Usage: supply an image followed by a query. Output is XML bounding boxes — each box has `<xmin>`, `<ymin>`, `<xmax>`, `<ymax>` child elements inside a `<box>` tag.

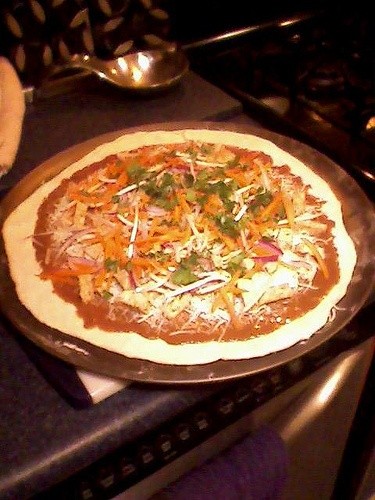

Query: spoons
<box><xmin>70</xmin><ymin>12</ymin><xmax>328</xmax><ymax>89</ymax></box>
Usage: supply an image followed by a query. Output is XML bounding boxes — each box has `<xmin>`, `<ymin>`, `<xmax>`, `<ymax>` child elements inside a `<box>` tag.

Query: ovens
<box><xmin>0</xmin><ymin>306</ymin><xmax>374</xmax><ymax>497</ymax></box>
